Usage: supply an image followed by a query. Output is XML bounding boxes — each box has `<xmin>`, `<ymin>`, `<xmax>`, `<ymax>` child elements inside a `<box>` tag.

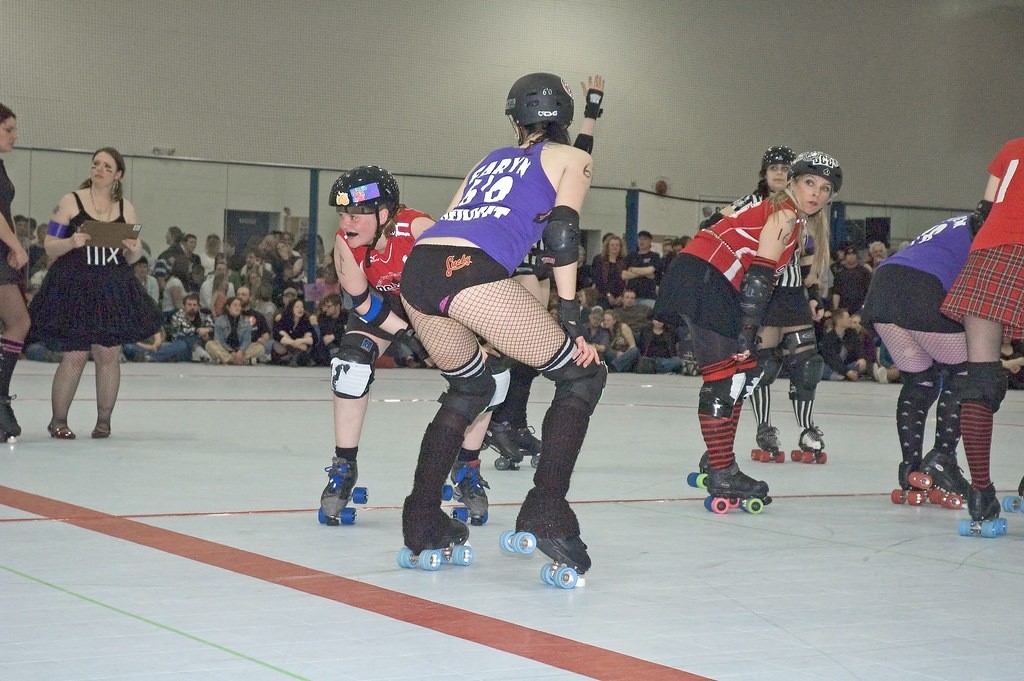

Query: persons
<box><xmin>940</xmin><ymin>138</ymin><xmax>1024</xmax><ymax>521</ymax></box>
<box><xmin>22</xmin><ymin>148</ymin><xmax>167</xmax><ymax>440</ymax></box>
<box><xmin>859</xmin><ymin>199</ymin><xmax>1024</xmax><ymax>503</ymax></box>
<box><xmin>0</xmin><ymin>103</ymin><xmax>31</xmax><ymax>443</ymax></box>
<box><xmin>650</xmin><ymin>146</ymin><xmax>842</xmax><ymax>499</ymax></box>
<box><xmin>398</xmin><ymin>73</ymin><xmax>609</xmax><ymax>575</ymax></box>
<box><xmin>13</xmin><ymin>214</ymin><xmax>1024</xmax><ymax>390</ymax></box>
<box><xmin>318</xmin><ymin>164</ymin><xmax>510</xmax><ymax>516</ymax></box>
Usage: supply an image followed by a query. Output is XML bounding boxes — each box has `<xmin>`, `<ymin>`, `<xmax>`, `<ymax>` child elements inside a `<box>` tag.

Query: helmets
<box><xmin>329</xmin><ymin>166</ymin><xmax>400</xmax><ymax>214</ymax></box>
<box><xmin>788</xmin><ymin>151</ymin><xmax>843</xmax><ymax>193</ymax></box>
<box><xmin>504</xmin><ymin>73</ymin><xmax>574</xmax><ymax>127</ymax></box>
<box><xmin>763</xmin><ymin>146</ymin><xmax>796</xmax><ymax>166</ymax></box>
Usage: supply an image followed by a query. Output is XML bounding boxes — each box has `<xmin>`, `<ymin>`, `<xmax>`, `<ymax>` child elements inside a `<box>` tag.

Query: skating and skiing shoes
<box><xmin>318</xmin><ymin>457</ymin><xmax>370</xmax><ymax>526</ymax></box>
<box><xmin>956</xmin><ymin>479</ymin><xmax>1024</xmax><ymax>537</ymax></box>
<box><xmin>498</xmin><ymin>495</ymin><xmax>593</xmax><ymax>590</ymax></box>
<box><xmin>686</xmin><ymin>451</ymin><xmax>773</xmax><ymax>514</ymax></box>
<box><xmin>750</xmin><ymin>423</ymin><xmax>785</xmax><ymax>464</ymax></box>
<box><xmin>480</xmin><ymin>419</ymin><xmax>545</xmax><ymax>471</ymax></box>
<box><xmin>442</xmin><ymin>459</ymin><xmax>491</xmax><ymax>526</ymax></box>
<box><xmin>791</xmin><ymin>427</ymin><xmax>829</xmax><ymax>465</ymax></box>
<box><xmin>398</xmin><ymin>496</ymin><xmax>476</xmax><ymax>572</ymax></box>
<box><xmin>890</xmin><ymin>449</ymin><xmax>970</xmax><ymax>511</ymax></box>
<box><xmin>0</xmin><ymin>395</ymin><xmax>22</xmax><ymax>443</ymax></box>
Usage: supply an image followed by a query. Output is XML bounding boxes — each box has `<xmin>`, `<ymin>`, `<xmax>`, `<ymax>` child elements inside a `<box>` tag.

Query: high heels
<box><xmin>47</xmin><ymin>421</ymin><xmax>76</xmax><ymax>439</ymax></box>
<box><xmin>91</xmin><ymin>420</ymin><xmax>112</xmax><ymax>438</ymax></box>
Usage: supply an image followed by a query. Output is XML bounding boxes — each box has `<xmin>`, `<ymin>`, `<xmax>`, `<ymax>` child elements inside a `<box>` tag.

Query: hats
<box><xmin>284</xmin><ymin>287</ymin><xmax>297</xmax><ymax>296</ymax></box>
<box><xmin>590</xmin><ymin>306</ymin><xmax>604</xmax><ymax>320</ymax></box>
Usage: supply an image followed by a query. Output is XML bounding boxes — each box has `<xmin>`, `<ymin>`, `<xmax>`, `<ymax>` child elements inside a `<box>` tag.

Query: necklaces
<box><xmin>90</xmin><ymin>186</ymin><xmax>113</xmax><ymax>222</ymax></box>
